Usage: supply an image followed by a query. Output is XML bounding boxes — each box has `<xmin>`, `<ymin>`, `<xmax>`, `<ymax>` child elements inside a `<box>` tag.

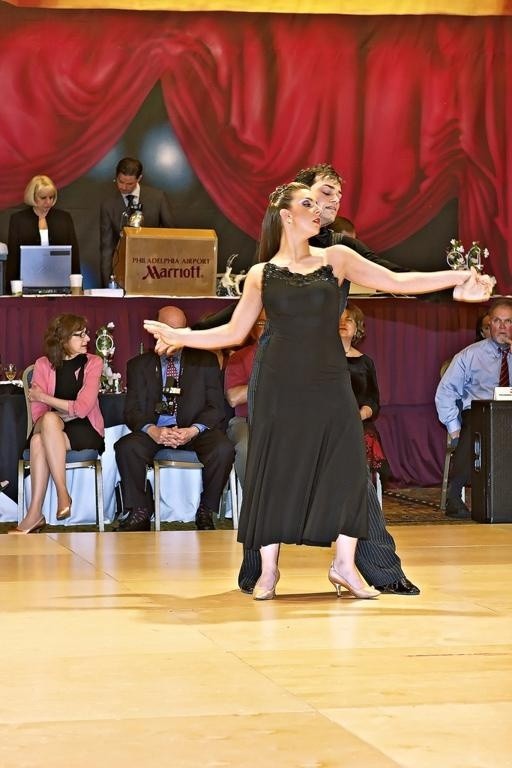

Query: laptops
<box><xmin>19</xmin><ymin>244</ymin><xmax>73</xmax><ymax>295</ymax></box>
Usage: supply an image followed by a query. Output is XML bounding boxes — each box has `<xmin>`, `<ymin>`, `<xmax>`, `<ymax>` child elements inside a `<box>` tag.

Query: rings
<box><xmin>28</xmin><ymin>394</ymin><xmax>31</xmax><ymax>399</ymax></box>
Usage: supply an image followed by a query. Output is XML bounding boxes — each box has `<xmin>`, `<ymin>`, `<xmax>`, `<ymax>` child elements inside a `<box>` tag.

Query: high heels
<box><xmin>7</xmin><ymin>514</ymin><xmax>46</xmax><ymax>535</ymax></box>
<box><xmin>56</xmin><ymin>496</ymin><xmax>72</xmax><ymax>520</ymax></box>
<box><xmin>328</xmin><ymin>560</ymin><xmax>381</xmax><ymax>599</ymax></box>
<box><xmin>252</xmin><ymin>565</ymin><xmax>280</xmax><ymax>600</ymax></box>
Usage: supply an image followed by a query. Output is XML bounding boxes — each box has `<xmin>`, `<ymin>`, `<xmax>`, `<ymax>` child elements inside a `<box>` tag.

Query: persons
<box><xmin>224</xmin><ymin>307</ymin><xmax>267</xmax><ymax>491</ymax></box>
<box><xmin>7</xmin><ymin>314</ymin><xmax>105</xmax><ymax>534</ymax></box>
<box><xmin>99</xmin><ymin>157</ymin><xmax>176</xmax><ymax>288</ymax></box>
<box><xmin>5</xmin><ymin>174</ymin><xmax>81</xmax><ymax>295</ymax></box>
<box><xmin>475</xmin><ymin>312</ymin><xmax>491</xmax><ymax>342</ymax></box>
<box><xmin>434</xmin><ymin>299</ymin><xmax>512</xmax><ymax>518</ymax></box>
<box><xmin>326</xmin><ymin>215</ymin><xmax>357</xmax><ymax>239</ymax></box>
<box><xmin>338</xmin><ymin>301</ymin><xmax>387</xmax><ymax>471</ymax></box>
<box><xmin>113</xmin><ymin>305</ymin><xmax>234</xmax><ymax>532</ymax></box>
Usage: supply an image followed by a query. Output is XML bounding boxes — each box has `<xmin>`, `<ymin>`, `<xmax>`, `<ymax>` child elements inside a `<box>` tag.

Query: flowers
<box><xmin>443</xmin><ymin>238</ymin><xmax>490</xmax><ymax>270</ymax></box>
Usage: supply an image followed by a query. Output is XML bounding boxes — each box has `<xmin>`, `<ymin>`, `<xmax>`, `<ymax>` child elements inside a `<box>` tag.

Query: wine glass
<box><xmin>3</xmin><ymin>362</ymin><xmax>18</xmax><ymax>382</ymax></box>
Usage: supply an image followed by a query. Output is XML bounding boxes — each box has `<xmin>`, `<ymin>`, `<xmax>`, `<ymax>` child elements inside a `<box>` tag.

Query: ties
<box><xmin>165</xmin><ymin>355</ymin><xmax>179</xmax><ymax>417</ymax></box>
<box><xmin>498</xmin><ymin>348</ymin><xmax>510</xmax><ymax>387</ymax></box>
<box><xmin>126</xmin><ymin>195</ymin><xmax>134</xmax><ymax>207</ymax></box>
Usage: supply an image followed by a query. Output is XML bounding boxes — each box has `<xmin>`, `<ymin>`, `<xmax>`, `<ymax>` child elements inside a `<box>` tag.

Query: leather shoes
<box><xmin>195</xmin><ymin>504</ymin><xmax>214</xmax><ymax>530</ymax></box>
<box><xmin>376</xmin><ymin>576</ymin><xmax>420</xmax><ymax>595</ymax></box>
<box><xmin>113</xmin><ymin>507</ymin><xmax>151</xmax><ymax>531</ymax></box>
<box><xmin>445</xmin><ymin>499</ymin><xmax>471</xmax><ymax>517</ymax></box>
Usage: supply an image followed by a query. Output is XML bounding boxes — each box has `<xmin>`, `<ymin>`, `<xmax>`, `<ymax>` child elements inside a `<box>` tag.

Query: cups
<box><xmin>68</xmin><ymin>273</ymin><xmax>84</xmax><ymax>296</ymax></box>
<box><xmin>10</xmin><ymin>280</ymin><xmax>23</xmax><ymax>297</ymax></box>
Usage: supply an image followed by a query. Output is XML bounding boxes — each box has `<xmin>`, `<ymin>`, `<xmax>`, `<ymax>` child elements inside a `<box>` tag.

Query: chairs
<box><xmin>440</xmin><ymin>360</ymin><xmax>459</xmax><ymax>510</ymax></box>
<box><xmin>18</xmin><ymin>365</ymin><xmax>104</xmax><ymax>531</ymax></box>
<box><xmin>144</xmin><ymin>450</ymin><xmax>239</xmax><ymax>530</ymax></box>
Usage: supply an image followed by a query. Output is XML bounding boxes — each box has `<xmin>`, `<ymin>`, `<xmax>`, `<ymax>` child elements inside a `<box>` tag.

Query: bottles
<box><xmin>108</xmin><ymin>274</ymin><xmax>118</xmax><ymax>289</ymax></box>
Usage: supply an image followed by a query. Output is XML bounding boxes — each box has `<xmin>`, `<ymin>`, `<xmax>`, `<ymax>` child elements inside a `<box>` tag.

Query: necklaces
<box><xmin>345</xmin><ymin>345</ymin><xmax>351</xmax><ymax>354</ymax></box>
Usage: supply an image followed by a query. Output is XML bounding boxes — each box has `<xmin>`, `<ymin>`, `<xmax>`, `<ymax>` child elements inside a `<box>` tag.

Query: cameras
<box><xmin>154</xmin><ymin>377</ymin><xmax>182</xmax><ymax>416</ymax></box>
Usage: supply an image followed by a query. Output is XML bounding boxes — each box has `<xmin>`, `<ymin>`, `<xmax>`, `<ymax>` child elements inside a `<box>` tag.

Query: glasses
<box><xmin>73</xmin><ymin>330</ymin><xmax>91</xmax><ymax>338</ymax></box>
